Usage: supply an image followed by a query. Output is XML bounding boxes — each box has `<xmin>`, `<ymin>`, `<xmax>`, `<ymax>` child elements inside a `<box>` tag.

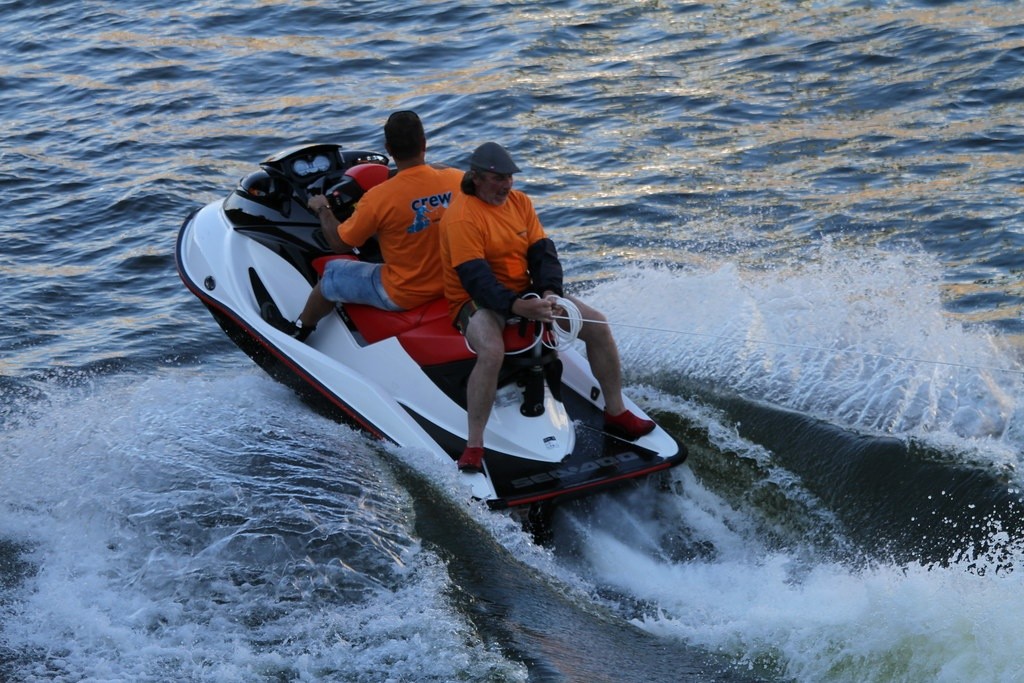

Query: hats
<box><xmin>471</xmin><ymin>142</ymin><xmax>522</xmax><ymax>174</ymax></box>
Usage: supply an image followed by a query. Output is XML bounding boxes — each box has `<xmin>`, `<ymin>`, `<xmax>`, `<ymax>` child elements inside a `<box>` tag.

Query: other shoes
<box><xmin>261</xmin><ymin>302</ymin><xmax>289</xmax><ymax>335</ymax></box>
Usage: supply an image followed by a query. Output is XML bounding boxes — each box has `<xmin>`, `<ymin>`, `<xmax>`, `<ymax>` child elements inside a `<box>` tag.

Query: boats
<box><xmin>175</xmin><ymin>142</ymin><xmax>689</xmax><ymax>511</ymax></box>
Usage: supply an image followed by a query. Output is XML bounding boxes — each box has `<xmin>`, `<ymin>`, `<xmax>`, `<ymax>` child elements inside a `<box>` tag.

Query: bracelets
<box><xmin>315</xmin><ymin>204</ymin><xmax>332</xmax><ymax>213</ymax></box>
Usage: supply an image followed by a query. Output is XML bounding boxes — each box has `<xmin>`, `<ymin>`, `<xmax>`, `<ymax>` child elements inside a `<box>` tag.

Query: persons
<box><xmin>260</xmin><ymin>111</ymin><xmax>657</xmax><ymax>472</ymax></box>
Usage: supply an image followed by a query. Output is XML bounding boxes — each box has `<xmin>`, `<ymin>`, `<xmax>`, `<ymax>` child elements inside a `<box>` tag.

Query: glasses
<box><xmin>388</xmin><ymin>110</ymin><xmax>419</xmax><ymax>121</ymax></box>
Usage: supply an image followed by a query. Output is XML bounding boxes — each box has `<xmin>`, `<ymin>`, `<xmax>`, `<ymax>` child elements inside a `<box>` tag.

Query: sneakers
<box><xmin>602</xmin><ymin>406</ymin><xmax>656</xmax><ymax>437</ymax></box>
<box><xmin>458</xmin><ymin>445</ymin><xmax>485</xmax><ymax>470</ymax></box>
